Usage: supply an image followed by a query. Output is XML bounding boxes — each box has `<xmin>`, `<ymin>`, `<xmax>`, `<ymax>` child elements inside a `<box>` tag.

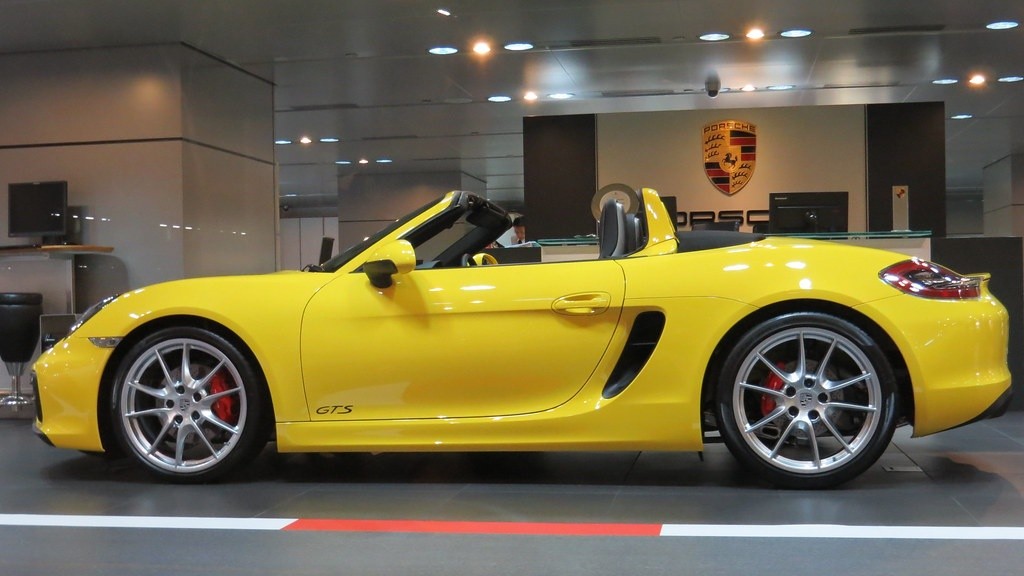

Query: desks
<box><xmin>0</xmin><ymin>245</ymin><xmax>114</xmax><ymax>314</ymax></box>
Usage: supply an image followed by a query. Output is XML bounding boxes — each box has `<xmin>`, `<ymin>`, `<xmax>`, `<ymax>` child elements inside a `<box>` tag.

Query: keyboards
<box><xmin>0</xmin><ymin>242</ymin><xmax>41</xmax><ymax>250</ymax></box>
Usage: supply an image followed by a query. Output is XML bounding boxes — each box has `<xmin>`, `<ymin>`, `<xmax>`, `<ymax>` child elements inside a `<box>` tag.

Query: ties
<box><xmin>518</xmin><ymin>240</ymin><xmax>522</xmax><ymax>244</ymax></box>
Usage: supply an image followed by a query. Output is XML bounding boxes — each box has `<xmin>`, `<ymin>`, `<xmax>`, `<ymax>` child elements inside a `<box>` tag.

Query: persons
<box><xmin>510</xmin><ymin>216</ymin><xmax>526</xmax><ymax>245</ymax></box>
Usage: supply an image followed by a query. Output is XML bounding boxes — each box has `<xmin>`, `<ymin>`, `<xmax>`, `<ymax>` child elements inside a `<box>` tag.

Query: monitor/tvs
<box><xmin>768</xmin><ymin>191</ymin><xmax>849</xmax><ymax>235</ymax></box>
<box><xmin>7</xmin><ymin>180</ymin><xmax>68</xmax><ymax>245</ymax></box>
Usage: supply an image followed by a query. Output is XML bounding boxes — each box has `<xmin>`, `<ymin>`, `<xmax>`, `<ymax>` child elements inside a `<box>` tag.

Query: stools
<box><xmin>0</xmin><ymin>292</ymin><xmax>44</xmax><ymax>412</ymax></box>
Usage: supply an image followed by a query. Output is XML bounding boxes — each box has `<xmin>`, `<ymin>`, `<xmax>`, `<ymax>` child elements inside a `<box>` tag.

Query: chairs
<box><xmin>625</xmin><ymin>213</ymin><xmax>644</xmax><ymax>254</ymax></box>
<box><xmin>597</xmin><ymin>198</ymin><xmax>629</xmax><ymax>258</ymax></box>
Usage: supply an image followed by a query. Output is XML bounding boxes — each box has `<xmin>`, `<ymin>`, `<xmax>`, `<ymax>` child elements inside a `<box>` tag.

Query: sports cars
<box><xmin>25</xmin><ymin>186</ymin><xmax>1014</xmax><ymax>492</ymax></box>
